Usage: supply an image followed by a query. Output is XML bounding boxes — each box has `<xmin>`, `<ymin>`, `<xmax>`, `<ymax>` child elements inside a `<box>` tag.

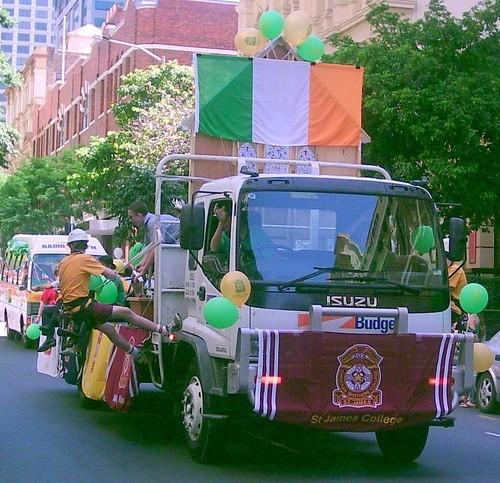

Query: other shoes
<box><xmin>57</xmin><ymin>328</ymin><xmax>89</xmax><ymax>359</ymax></box>
<box><xmin>37</xmin><ymin>338</ymin><xmax>55</xmax><ymax>352</ymax></box>
<box><xmin>39</xmin><ymin>326</ymin><xmax>54</xmax><ymax>338</ymax></box>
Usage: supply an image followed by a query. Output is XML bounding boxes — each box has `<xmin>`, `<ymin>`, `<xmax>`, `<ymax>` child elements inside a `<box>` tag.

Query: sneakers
<box><xmin>166</xmin><ymin>312</ymin><xmax>182</xmax><ymax>337</ymax></box>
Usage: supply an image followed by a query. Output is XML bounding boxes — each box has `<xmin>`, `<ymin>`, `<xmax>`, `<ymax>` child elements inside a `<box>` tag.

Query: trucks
<box><xmin>52</xmin><ymin>153</ymin><xmax>477</xmax><ymax>466</ymax></box>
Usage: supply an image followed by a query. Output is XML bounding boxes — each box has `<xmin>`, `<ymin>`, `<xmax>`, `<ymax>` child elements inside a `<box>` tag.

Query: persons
<box><xmin>448</xmin><ymin>264</ymin><xmax>468</xmax><ymax>326</ymax></box>
<box><xmin>127</xmin><ymin>201</ymin><xmax>182</xmax><ymax>282</ymax></box>
<box><xmin>22</xmin><ymin>267</ymin><xmax>47</xmax><ymax>285</ymax></box>
<box><xmin>210</xmin><ymin>209</ymin><xmax>259</xmax><ymax>267</ymax></box>
<box><xmin>35</xmin><ymin>228</ymin><xmax>183</xmax><ymax>363</ymax></box>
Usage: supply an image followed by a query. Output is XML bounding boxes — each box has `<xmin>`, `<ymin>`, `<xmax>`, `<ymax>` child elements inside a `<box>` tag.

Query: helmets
<box><xmin>67</xmin><ymin>229</ymin><xmax>89</xmax><ymax>244</ymax></box>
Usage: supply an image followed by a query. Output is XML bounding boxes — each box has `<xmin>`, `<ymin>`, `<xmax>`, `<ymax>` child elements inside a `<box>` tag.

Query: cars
<box><xmin>476</xmin><ymin>329</ymin><xmax>500</xmax><ymax>413</ymax></box>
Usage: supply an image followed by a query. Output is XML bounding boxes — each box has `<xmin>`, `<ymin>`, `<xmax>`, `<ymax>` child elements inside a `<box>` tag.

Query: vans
<box><xmin>1</xmin><ymin>234</ymin><xmax>111</xmax><ymax>348</ymax></box>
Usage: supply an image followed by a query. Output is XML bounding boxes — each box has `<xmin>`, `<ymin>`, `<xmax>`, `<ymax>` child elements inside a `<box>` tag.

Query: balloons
<box><xmin>283</xmin><ymin>10</ymin><xmax>312</xmax><ymax>46</ymax></box>
<box><xmin>259</xmin><ymin>10</ymin><xmax>284</xmax><ymax>40</ymax></box>
<box><xmin>297</xmin><ymin>36</ymin><xmax>324</xmax><ymax>61</ymax></box>
<box><xmin>114</xmin><ymin>248</ymin><xmax>123</xmax><ymax>259</ymax></box>
<box><xmin>26</xmin><ymin>324</ymin><xmax>41</xmax><ymax>340</ymax></box>
<box><xmin>459</xmin><ymin>283</ymin><xmax>488</xmax><ymax>314</ymax></box>
<box><xmin>234</xmin><ymin>28</ymin><xmax>267</xmax><ymax>56</ymax></box>
<box><xmin>220</xmin><ymin>270</ymin><xmax>251</xmax><ymax>309</ymax></box>
<box><xmin>129</xmin><ymin>243</ymin><xmax>146</xmax><ymax>265</ymax></box>
<box><xmin>409</xmin><ymin>226</ymin><xmax>435</xmax><ymax>255</ymax></box>
<box><xmin>203</xmin><ymin>298</ymin><xmax>239</xmax><ymax>329</ymax></box>
<box><xmin>96</xmin><ymin>280</ymin><xmax>118</xmax><ymax>304</ymax></box>
<box><xmin>88</xmin><ymin>275</ymin><xmax>103</xmax><ymax>295</ymax></box>
<box><xmin>474</xmin><ymin>342</ymin><xmax>495</xmax><ymax>372</ymax></box>
<box><xmin>7</xmin><ymin>239</ymin><xmax>30</xmax><ymax>256</ymax></box>
